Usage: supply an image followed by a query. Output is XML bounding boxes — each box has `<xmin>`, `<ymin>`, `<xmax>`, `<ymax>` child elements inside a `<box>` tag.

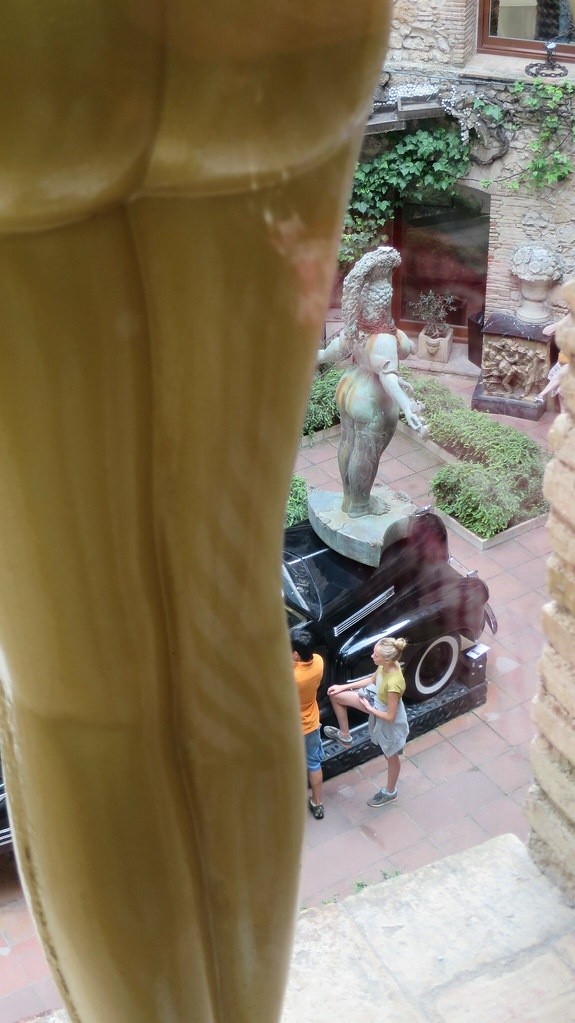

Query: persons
<box><xmin>481</xmin><ymin>339</ymin><xmax>547</xmax><ymax>398</ymax></box>
<box><xmin>318</xmin><ymin>246</ymin><xmax>423</xmax><ymax>518</ymax></box>
<box><xmin>288</xmin><ymin>628</ymin><xmax>324</xmax><ymax>819</ymax></box>
<box><xmin>324</xmin><ymin>637</ymin><xmax>409</xmax><ymax>808</ymax></box>
<box><xmin>533</xmin><ymin>361</ymin><xmax>568</xmax><ymax>414</ymax></box>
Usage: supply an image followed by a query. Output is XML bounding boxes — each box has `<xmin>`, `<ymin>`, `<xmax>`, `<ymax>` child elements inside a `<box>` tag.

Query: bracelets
<box><xmin>347</xmin><ymin>684</ymin><xmax>354</xmax><ymax>692</ymax></box>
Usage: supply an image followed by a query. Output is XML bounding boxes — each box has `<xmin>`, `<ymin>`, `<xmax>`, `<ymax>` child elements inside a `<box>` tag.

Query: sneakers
<box><xmin>324</xmin><ymin>726</ymin><xmax>354</xmax><ymax>750</ymax></box>
<box><xmin>307</xmin><ymin>796</ymin><xmax>324</xmax><ymax>819</ymax></box>
<box><xmin>367</xmin><ymin>786</ymin><xmax>398</xmax><ymax>808</ymax></box>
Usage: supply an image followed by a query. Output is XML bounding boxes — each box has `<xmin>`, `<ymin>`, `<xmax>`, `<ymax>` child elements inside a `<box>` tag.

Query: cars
<box><xmin>0</xmin><ymin>501</ymin><xmax>498</xmax><ymax>839</ymax></box>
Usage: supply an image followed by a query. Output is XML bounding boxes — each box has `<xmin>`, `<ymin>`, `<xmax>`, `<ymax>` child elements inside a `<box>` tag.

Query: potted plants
<box><xmin>408</xmin><ymin>288</ymin><xmax>461</xmax><ymax>364</ymax></box>
